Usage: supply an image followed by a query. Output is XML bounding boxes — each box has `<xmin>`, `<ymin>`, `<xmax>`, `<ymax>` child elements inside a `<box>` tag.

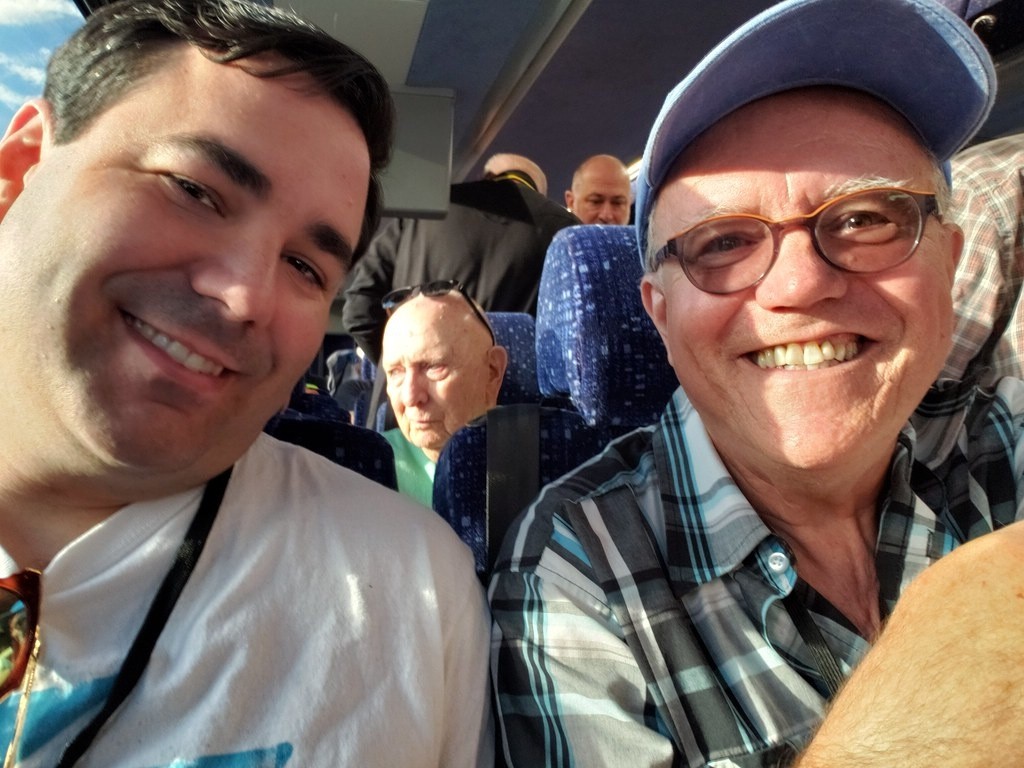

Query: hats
<box><xmin>634</xmin><ymin>0</ymin><xmax>997</xmax><ymax>270</ymax></box>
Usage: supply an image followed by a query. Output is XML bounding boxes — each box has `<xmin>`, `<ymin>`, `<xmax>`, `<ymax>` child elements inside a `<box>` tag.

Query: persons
<box><xmin>565</xmin><ymin>154</ymin><xmax>632</xmax><ymax>227</ymax></box>
<box><xmin>1</xmin><ymin>0</ymin><xmax>495</xmax><ymax>767</ymax></box>
<box><xmin>487</xmin><ymin>0</ymin><xmax>1024</xmax><ymax>767</ymax></box>
<box><xmin>343</xmin><ymin>154</ymin><xmax>583</xmax><ymax>432</ymax></box>
<box><xmin>380</xmin><ymin>291</ymin><xmax>507</xmax><ymax>507</ymax></box>
<box><xmin>304</xmin><ymin>366</ymin><xmax>374</xmax><ymax>426</ymax></box>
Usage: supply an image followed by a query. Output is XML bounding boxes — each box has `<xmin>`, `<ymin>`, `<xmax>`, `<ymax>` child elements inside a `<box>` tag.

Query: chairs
<box><xmin>269</xmin><ymin>221</ymin><xmax>676</xmax><ymax>579</ymax></box>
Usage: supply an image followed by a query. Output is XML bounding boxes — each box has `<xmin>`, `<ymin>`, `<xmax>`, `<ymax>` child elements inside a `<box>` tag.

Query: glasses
<box><xmin>0</xmin><ymin>568</ymin><xmax>42</xmax><ymax>703</ymax></box>
<box><xmin>649</xmin><ymin>186</ymin><xmax>943</xmax><ymax>296</ymax></box>
<box><xmin>382</xmin><ymin>279</ymin><xmax>495</xmax><ymax>346</ymax></box>
